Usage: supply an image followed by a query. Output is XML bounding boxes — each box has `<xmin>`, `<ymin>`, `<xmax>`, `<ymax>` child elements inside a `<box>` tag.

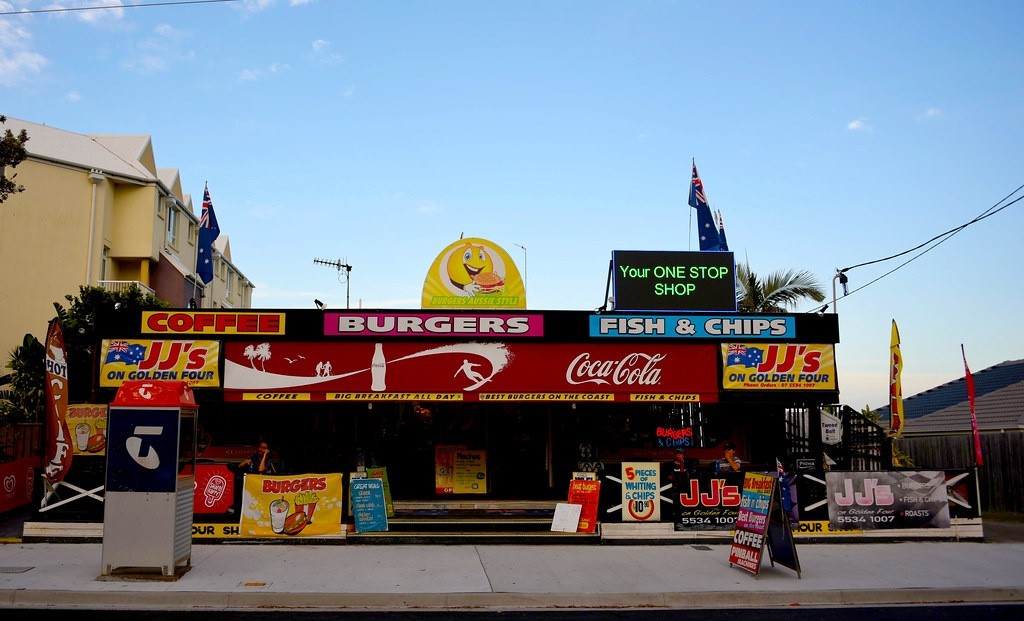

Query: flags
<box><xmin>963</xmin><ymin>349</ymin><xmax>984</xmax><ymax>464</ymax></box>
<box><xmin>686</xmin><ymin>165</ymin><xmax>729</xmax><ymax>252</ymax></box>
<box><xmin>45</xmin><ymin>321</ymin><xmax>74</xmax><ymax>481</ymax></box>
<box><xmin>889</xmin><ymin>322</ymin><xmax>902</xmax><ymax>438</ymax></box>
<box><xmin>195</xmin><ymin>188</ymin><xmax>221</xmax><ymax>285</ymax></box>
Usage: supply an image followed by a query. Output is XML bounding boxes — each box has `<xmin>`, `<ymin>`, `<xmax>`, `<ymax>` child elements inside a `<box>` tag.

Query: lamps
<box><xmin>594</xmin><ymin>305</ymin><xmax>607</xmax><ymax>315</ymax></box>
<box><xmin>816</xmin><ymin>304</ymin><xmax>829</xmax><ymax>317</ymax></box>
<box><xmin>314</xmin><ymin>299</ymin><xmax>327</xmax><ymax>310</ymax></box>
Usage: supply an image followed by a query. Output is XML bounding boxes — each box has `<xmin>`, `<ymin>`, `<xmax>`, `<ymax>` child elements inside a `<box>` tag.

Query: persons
<box><xmin>669</xmin><ymin>445</ymin><xmax>741</xmax><ymax>473</ymax></box>
<box><xmin>236</xmin><ymin>441</ymin><xmax>273</xmax><ymax>474</ymax></box>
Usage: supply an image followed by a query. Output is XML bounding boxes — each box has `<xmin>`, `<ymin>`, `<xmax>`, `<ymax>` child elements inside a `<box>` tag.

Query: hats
<box><xmin>673</xmin><ymin>445</ymin><xmax>687</xmax><ymax>455</ymax></box>
<box><xmin>722</xmin><ymin>442</ymin><xmax>737</xmax><ymax>450</ymax></box>
